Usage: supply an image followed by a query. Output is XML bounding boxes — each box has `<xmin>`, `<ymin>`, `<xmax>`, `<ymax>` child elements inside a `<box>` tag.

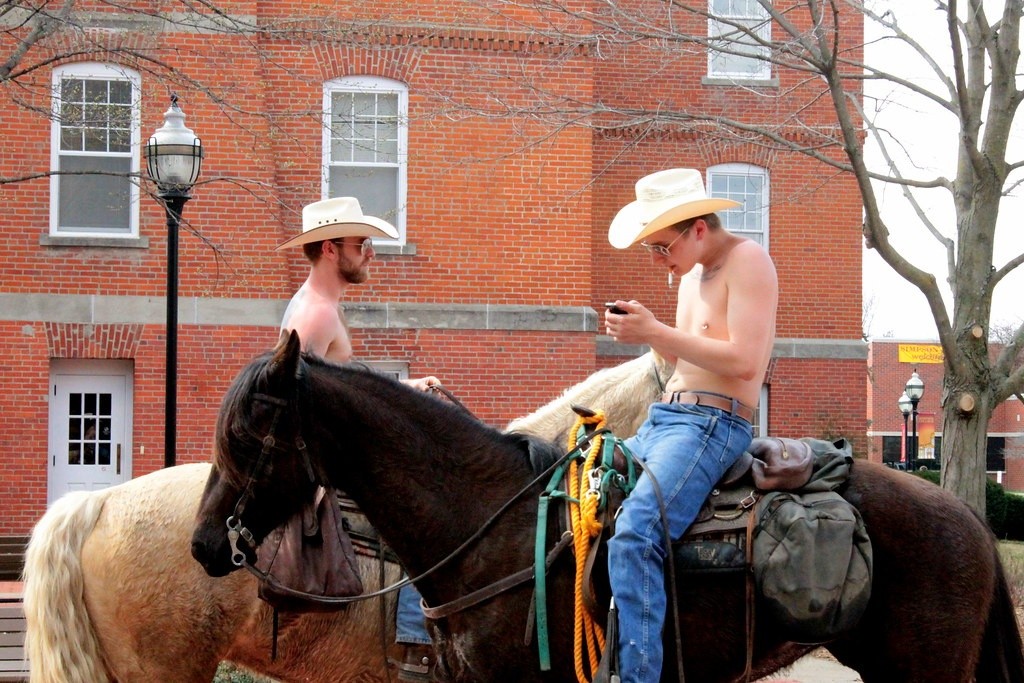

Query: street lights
<box><xmin>898</xmin><ymin>389</ymin><xmax>914</xmax><ymax>471</ymax></box>
<box><xmin>142</xmin><ymin>90</ymin><xmax>205</xmax><ymax>467</ymax></box>
<box><xmin>903</xmin><ymin>368</ymin><xmax>926</xmax><ymax>472</ymax></box>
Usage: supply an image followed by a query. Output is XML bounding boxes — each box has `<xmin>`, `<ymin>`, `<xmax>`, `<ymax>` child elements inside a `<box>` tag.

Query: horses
<box><xmin>19</xmin><ymin>342</ymin><xmax>679</xmax><ymax>682</ymax></box>
<box><xmin>190</xmin><ymin>327</ymin><xmax>1024</xmax><ymax>683</ymax></box>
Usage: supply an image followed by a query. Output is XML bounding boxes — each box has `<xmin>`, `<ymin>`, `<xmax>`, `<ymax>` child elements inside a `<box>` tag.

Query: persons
<box><xmin>275</xmin><ymin>197</ymin><xmax>445</xmax><ymax>683</ymax></box>
<box><xmin>604</xmin><ymin>168</ymin><xmax>780</xmax><ymax>683</ymax></box>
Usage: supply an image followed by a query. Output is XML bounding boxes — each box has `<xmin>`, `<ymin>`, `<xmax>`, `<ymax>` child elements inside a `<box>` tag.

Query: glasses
<box><xmin>641</xmin><ymin>227</ymin><xmax>689</xmax><ymax>256</ymax></box>
<box><xmin>335</xmin><ymin>238</ymin><xmax>372</xmax><ymax>256</ymax></box>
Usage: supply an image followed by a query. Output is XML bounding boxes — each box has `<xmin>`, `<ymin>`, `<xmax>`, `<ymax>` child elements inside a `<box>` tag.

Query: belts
<box><xmin>662</xmin><ymin>393</ymin><xmax>753</xmax><ymax>424</ymax></box>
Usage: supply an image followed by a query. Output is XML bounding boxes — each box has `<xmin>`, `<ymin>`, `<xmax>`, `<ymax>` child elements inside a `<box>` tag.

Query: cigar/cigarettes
<box><xmin>668</xmin><ymin>266</ymin><xmax>673</xmax><ymax>288</ymax></box>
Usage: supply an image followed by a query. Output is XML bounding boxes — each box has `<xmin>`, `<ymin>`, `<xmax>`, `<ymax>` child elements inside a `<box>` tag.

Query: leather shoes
<box><xmin>398</xmin><ymin>643</ymin><xmax>435</xmax><ymax>683</ymax></box>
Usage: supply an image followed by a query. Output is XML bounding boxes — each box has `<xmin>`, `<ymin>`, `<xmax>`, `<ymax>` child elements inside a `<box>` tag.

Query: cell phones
<box><xmin>605</xmin><ymin>303</ymin><xmax>628</xmax><ymax>314</ymax></box>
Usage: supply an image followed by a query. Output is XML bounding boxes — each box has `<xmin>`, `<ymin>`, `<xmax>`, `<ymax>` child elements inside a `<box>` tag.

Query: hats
<box><xmin>273</xmin><ymin>197</ymin><xmax>400</xmax><ymax>251</ymax></box>
<box><xmin>608</xmin><ymin>168</ymin><xmax>743</xmax><ymax>249</ymax></box>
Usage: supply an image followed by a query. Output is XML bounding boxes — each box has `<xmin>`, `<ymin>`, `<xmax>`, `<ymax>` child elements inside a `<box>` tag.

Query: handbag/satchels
<box><xmin>745</xmin><ymin>491</ymin><xmax>873</xmax><ymax>644</ymax></box>
<box><xmin>258</xmin><ymin>488</ymin><xmax>364</xmax><ymax>612</ymax></box>
